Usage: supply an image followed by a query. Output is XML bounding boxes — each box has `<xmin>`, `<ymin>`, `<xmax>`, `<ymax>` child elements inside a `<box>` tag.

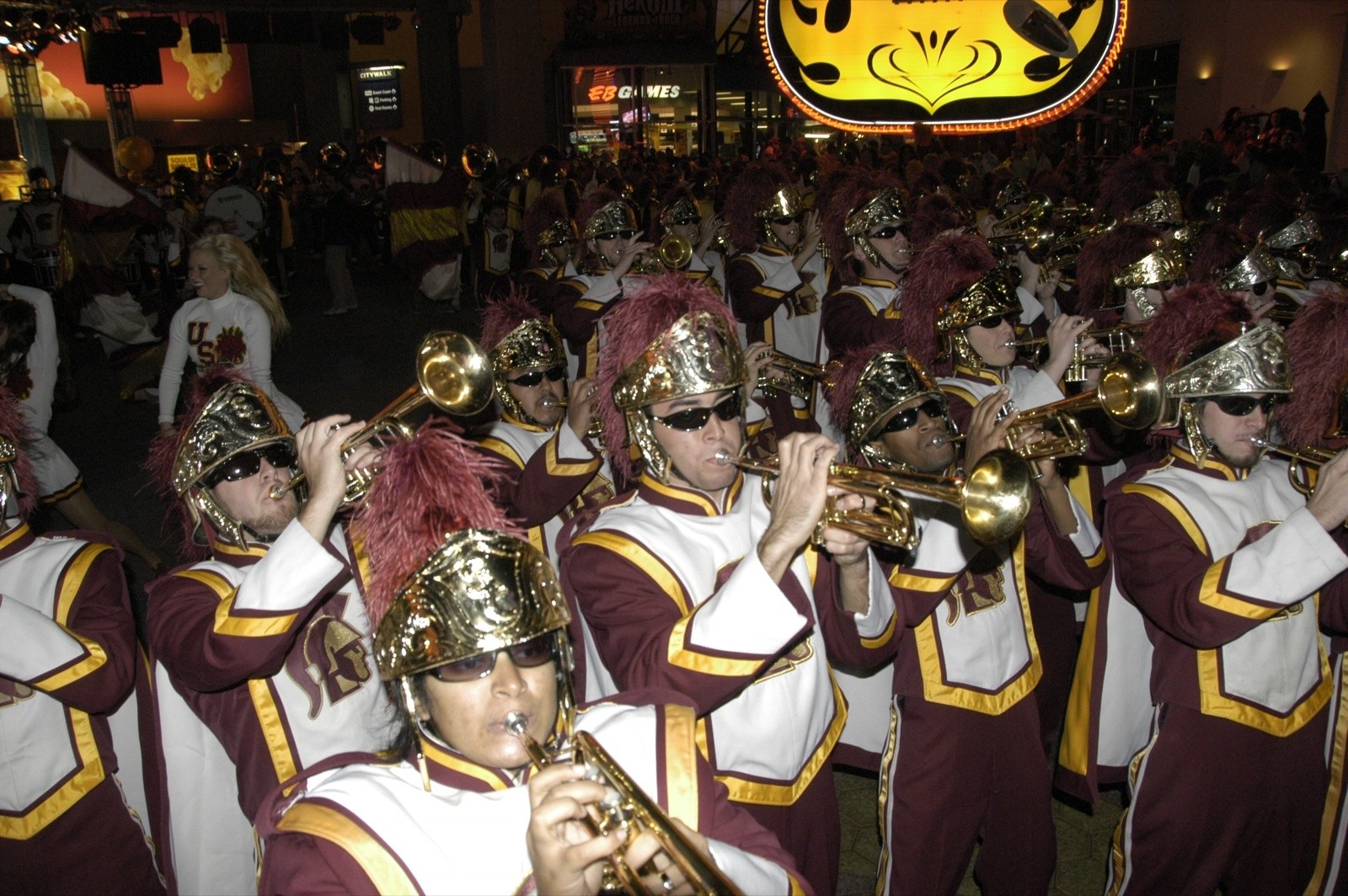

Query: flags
<box><xmin>386</xmin><ymin>144</ymin><xmax>481</xmax><ymax>304</ymax></box>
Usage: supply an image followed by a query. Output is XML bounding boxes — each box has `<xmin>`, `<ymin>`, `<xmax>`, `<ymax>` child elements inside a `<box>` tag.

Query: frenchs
<box><xmin>461</xmin><ymin>139</ymin><xmax>499</xmax><ymax>178</ymax></box>
<box><xmin>359</xmin><ymin>139</ymin><xmax>387</xmax><ymax>172</ymax></box>
<box><xmin>416</xmin><ymin>138</ymin><xmax>448</xmax><ymax>172</ymax></box>
<box><xmin>200</xmin><ymin>141</ymin><xmax>247</xmax><ymax>184</ymax></box>
<box><xmin>318</xmin><ymin>140</ymin><xmax>350</xmax><ymax>173</ymax></box>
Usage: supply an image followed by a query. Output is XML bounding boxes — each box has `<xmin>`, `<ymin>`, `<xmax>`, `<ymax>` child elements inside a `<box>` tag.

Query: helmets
<box><xmin>902</xmin><ymin>227</ymin><xmax>1025</xmax><ymax>371</ymax></box>
<box><xmin>829</xmin><ymin>342</ymin><xmax>962</xmax><ymax>473</ymax></box>
<box><xmin>350</xmin><ymin>423</ymin><xmax>570</xmax><ymax>735</ymax></box>
<box><xmin>597</xmin><ymin>269</ymin><xmax>749</xmax><ymax>484</ymax></box>
<box><xmin>1157</xmin><ymin>292</ymin><xmax>1293</xmax><ymax>469</ymax></box>
<box><xmin>528</xmin><ymin>160</ymin><xmax>806</xmax><ymax>272</ymax></box>
<box><xmin>828</xmin><ymin>169</ymin><xmax>906</xmax><ymax>269</ymax></box>
<box><xmin>484</xmin><ymin>299</ymin><xmax>569</xmax><ymax>416</ymax></box>
<box><xmin>993</xmin><ymin>157</ymin><xmax>1327</xmax><ymax>315</ymax></box>
<box><xmin>147</xmin><ymin>364</ymin><xmax>298</xmax><ymax>549</ymax></box>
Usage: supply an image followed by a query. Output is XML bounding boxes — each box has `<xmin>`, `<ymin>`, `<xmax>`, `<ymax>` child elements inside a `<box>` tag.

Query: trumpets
<box><xmin>635</xmin><ymin>232</ymin><xmax>693</xmax><ymax>272</ymax></box>
<box><xmin>1246</xmin><ymin>431</ymin><xmax>1340</xmax><ymax>498</ymax></box>
<box><xmin>503</xmin><ymin>708</ymin><xmax>745</xmax><ymax>896</ymax></box>
<box><xmin>714</xmin><ymin>351</ymin><xmax>1164</xmax><ymax>550</ymax></box>
<box><xmin>791</xmin><ymin>219</ymin><xmax>832</xmax><ymax>259</ymax></box>
<box><xmin>542</xmin><ymin>393</ymin><xmax>604</xmax><ymax>437</ymax></box>
<box><xmin>268</xmin><ymin>329</ymin><xmax>497</xmax><ymax>500</ymax></box>
<box><xmin>702</xmin><ymin>218</ymin><xmax>734</xmax><ymax>248</ymax></box>
<box><xmin>898</xmin><ymin>198</ymin><xmax>1348</xmax><ymax>383</ymax></box>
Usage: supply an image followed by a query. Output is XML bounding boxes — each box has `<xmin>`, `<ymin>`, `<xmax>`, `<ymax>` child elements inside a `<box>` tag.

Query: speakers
<box><xmin>79</xmin><ymin>32</ymin><xmax>163</xmax><ymax>85</ymax></box>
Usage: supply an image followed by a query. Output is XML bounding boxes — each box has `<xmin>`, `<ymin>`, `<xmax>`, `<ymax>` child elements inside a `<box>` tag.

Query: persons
<box><xmin>908</xmin><ymin>122</ymin><xmax>1116</xmax><ymax>762</ymax></box>
<box><xmin>0</xmin><ymin>143</ymin><xmax>385</xmax><ymax>895</ymax></box>
<box><xmin>559</xmin><ymin>275</ymin><xmax>899</xmax><ymax>896</ymax></box>
<box><xmin>407</xmin><ymin>129</ymin><xmax>911</xmax><ymax>574</ymax></box>
<box><xmin>247</xmin><ymin>415</ymin><xmax>815</xmax><ymax>895</ymax></box>
<box><xmin>833</xmin><ymin>340</ymin><xmax>1109</xmax><ymax>895</ymax></box>
<box><xmin>1055</xmin><ymin>110</ymin><xmax>1347</xmax><ymax>896</ymax></box>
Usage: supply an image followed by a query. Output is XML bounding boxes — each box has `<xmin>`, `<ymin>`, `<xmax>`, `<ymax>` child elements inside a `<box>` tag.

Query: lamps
<box><xmin>1273</xmin><ymin>67</ymin><xmax>1287</xmax><ymax>77</ymax></box>
<box><xmin>1198</xmin><ymin>76</ymin><xmax>1207</xmax><ymax>85</ymax></box>
<box><xmin>0</xmin><ymin>13</ymin><xmax>91</xmax><ymax>54</ymax></box>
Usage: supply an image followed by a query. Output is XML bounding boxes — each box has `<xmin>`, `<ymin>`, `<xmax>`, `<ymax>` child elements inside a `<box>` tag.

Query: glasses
<box><xmin>1005</xmin><ymin>196</ymin><xmax>1029</xmax><ymax>205</ymax></box>
<box><xmin>672</xmin><ymin>216</ymin><xmax>701</xmax><ymax>225</ymax></box>
<box><xmin>872</xmin><ymin>399</ymin><xmax>943</xmax><ymax>442</ymax></box>
<box><xmin>203</xmin><ymin>443</ymin><xmax>293</xmax><ymax>490</ymax></box>
<box><xmin>1158</xmin><ymin>223</ymin><xmax>1175</xmax><ymax>231</ymax></box>
<box><xmin>595</xmin><ymin>230</ymin><xmax>632</xmax><ymax>240</ymax></box>
<box><xmin>430</xmin><ymin>635</ymin><xmax>554</xmax><ymax>683</ymax></box>
<box><xmin>1202</xmin><ymin>395</ymin><xmax>1273</xmax><ymax>416</ymax></box>
<box><xmin>1252</xmin><ymin>278</ymin><xmax>1277</xmax><ymax>296</ymax></box>
<box><xmin>549</xmin><ymin>239</ymin><xmax>573</xmax><ymax>246</ymax></box>
<box><xmin>1144</xmin><ymin>278</ymin><xmax>1184</xmax><ymax>291</ymax></box>
<box><xmin>772</xmin><ymin>216</ymin><xmax>800</xmax><ymax>225</ymax></box>
<box><xmin>869</xmin><ymin>225</ymin><xmax>909</xmax><ymax>238</ymax></box>
<box><xmin>966</xmin><ymin>312</ymin><xmax>1014</xmax><ymax>330</ymax></box>
<box><xmin>647</xmin><ymin>395</ymin><xmax>737</xmax><ymax>432</ymax></box>
<box><xmin>506</xmin><ymin>368</ymin><xmax>564</xmax><ymax>386</ymax></box>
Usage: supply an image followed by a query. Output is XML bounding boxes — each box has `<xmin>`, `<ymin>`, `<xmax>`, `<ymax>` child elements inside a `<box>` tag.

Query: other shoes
<box><xmin>323</xmin><ymin>307</ymin><xmax>348</xmax><ymax>316</ymax></box>
<box><xmin>347</xmin><ymin>303</ymin><xmax>358</xmax><ymax>309</ymax></box>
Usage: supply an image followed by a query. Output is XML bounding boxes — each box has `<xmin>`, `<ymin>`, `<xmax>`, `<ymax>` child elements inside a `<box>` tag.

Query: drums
<box><xmin>202</xmin><ymin>187</ymin><xmax>268</xmax><ymax>248</ymax></box>
